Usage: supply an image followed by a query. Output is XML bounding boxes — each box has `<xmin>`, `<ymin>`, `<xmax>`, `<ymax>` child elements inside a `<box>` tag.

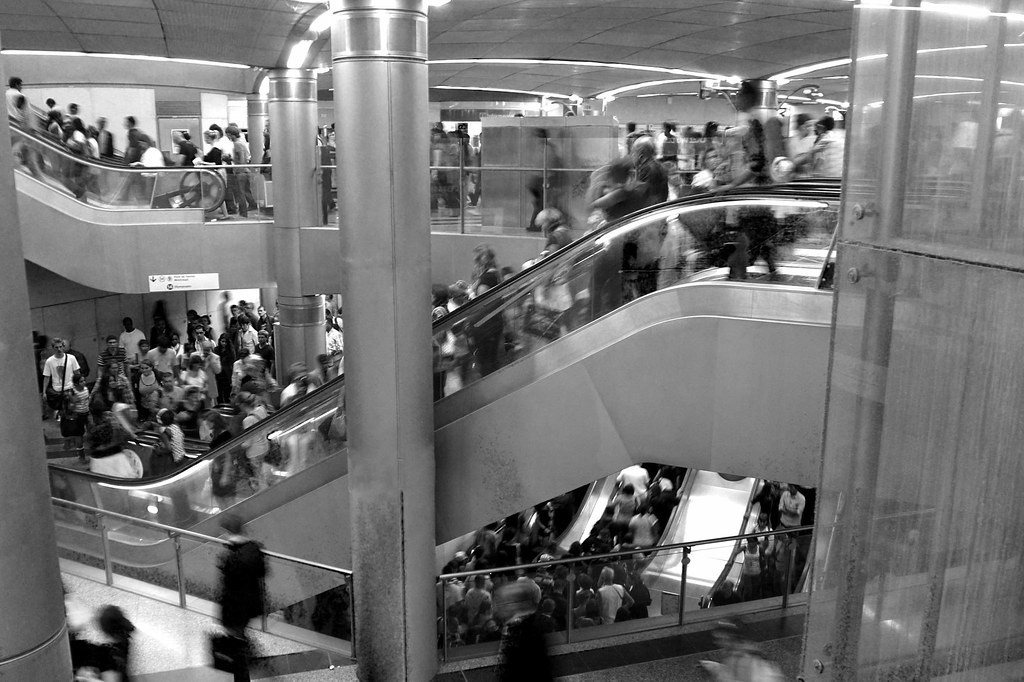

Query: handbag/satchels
<box><xmin>59</xmin><ymin>409</ymin><xmax>85</xmax><ymax>438</ymax></box>
<box><xmin>522</xmin><ymin>304</ymin><xmax>563</xmax><ymax>341</ymax></box>
<box><xmin>430</xmin><ymin>306</ymin><xmax>457</xmax><ymax>357</ymax></box>
<box><xmin>327</xmin><ymin>385</ymin><xmax>348</xmax><ymax>441</ymax></box>
<box><xmin>210</xmin><ymin>631</ymin><xmax>249</xmax><ymax>675</ymax></box>
<box><xmin>151</xmin><ymin>448</ymin><xmax>174</xmax><ymax>476</ymax></box>
<box><xmin>264</xmin><ymin>439</ymin><xmax>282</xmax><ymax>467</ymax></box>
<box><xmin>46</xmin><ymin>386</ymin><xmax>64</xmax><ymax>411</ymax></box>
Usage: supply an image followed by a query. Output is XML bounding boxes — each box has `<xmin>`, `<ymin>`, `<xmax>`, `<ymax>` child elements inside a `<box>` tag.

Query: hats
<box><xmin>535</xmin><ymin>208</ymin><xmax>564</xmax><ymax>237</ymax></box>
<box><xmin>455</xmin><ymin>551</ymin><xmax>468</xmax><ymax>561</ymax></box>
<box><xmin>771</xmin><ymin>156</ymin><xmax>798</xmax><ymax>183</ymax></box>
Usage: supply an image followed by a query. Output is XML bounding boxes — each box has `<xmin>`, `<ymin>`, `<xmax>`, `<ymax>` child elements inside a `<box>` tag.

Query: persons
<box><xmin>2</xmin><ymin>77</ymin><xmax>1024</xmax><ymax>682</ymax></box>
<box><xmin>213</xmin><ymin>514</ymin><xmax>267</xmax><ymax>682</ymax></box>
<box><xmin>490</xmin><ymin>581</ymin><xmax>553</xmax><ymax>681</ymax></box>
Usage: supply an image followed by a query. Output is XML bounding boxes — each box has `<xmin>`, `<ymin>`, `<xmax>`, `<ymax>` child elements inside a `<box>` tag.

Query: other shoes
<box><xmin>233</xmin><ymin>215</ymin><xmax>246</xmax><ymax>221</ymax></box>
<box><xmin>247</xmin><ymin>206</ymin><xmax>257</xmax><ymax>211</ymax></box>
<box><xmin>526</xmin><ymin>226</ymin><xmax>542</xmax><ymax>232</ymax></box>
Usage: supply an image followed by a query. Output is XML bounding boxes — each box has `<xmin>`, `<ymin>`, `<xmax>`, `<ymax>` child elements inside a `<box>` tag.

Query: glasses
<box><xmin>195</xmin><ymin>331</ymin><xmax>203</xmax><ymax>335</ymax></box>
